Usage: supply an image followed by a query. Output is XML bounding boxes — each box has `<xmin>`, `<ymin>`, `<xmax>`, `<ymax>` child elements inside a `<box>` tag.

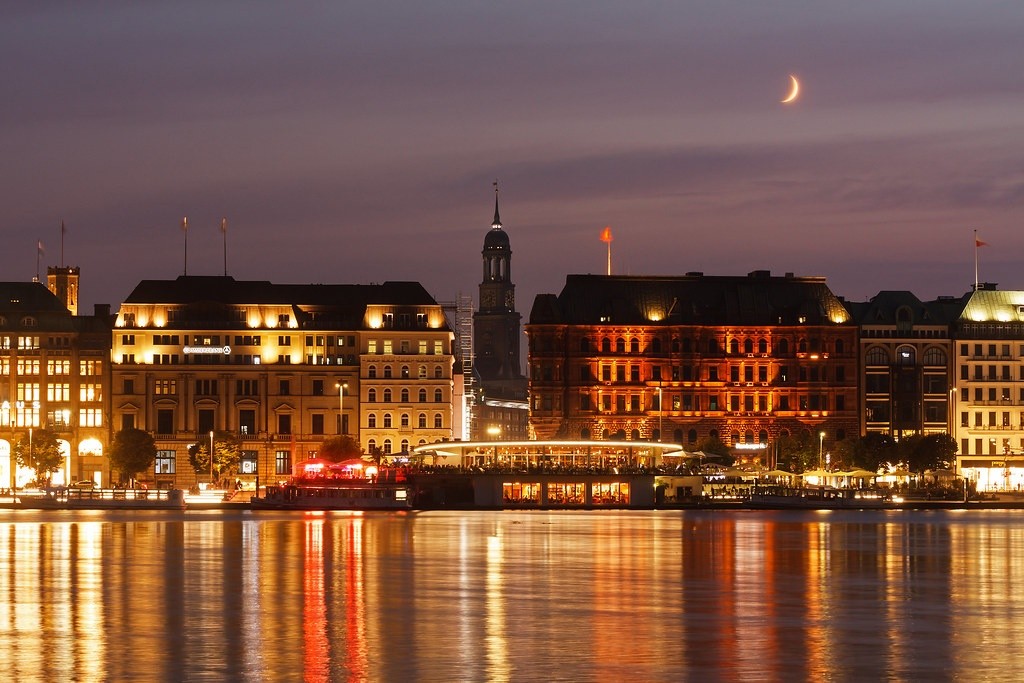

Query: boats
<box><xmin>742</xmin><ymin>485</ymin><xmax>898</xmax><ymax>509</ymax></box>
<box><xmin>249</xmin><ymin>475</ymin><xmax>417</xmax><ymax>510</ymax></box>
<box><xmin>19</xmin><ymin>488</ymin><xmax>189</xmax><ymax>510</ymax></box>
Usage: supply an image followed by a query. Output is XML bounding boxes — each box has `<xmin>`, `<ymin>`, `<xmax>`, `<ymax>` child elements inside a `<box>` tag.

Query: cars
<box><xmin>112</xmin><ymin>479</ymin><xmax>147</xmax><ymax>489</ymax></box>
<box><xmin>69</xmin><ymin>480</ymin><xmax>97</xmax><ymax>489</ymax></box>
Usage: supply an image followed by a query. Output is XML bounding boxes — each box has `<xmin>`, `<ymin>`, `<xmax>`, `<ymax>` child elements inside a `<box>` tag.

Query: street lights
<box><xmin>819</xmin><ymin>432</ymin><xmax>826</xmax><ymax>486</ymax></box>
<box><xmin>655</xmin><ymin>387</ymin><xmax>662</xmax><ymax>443</ymax></box>
<box><xmin>335</xmin><ymin>382</ymin><xmax>348</xmax><ymax>435</ymax></box>
<box><xmin>209</xmin><ymin>430</ymin><xmax>214</xmax><ymax>498</ymax></box>
<box><xmin>949</xmin><ymin>387</ymin><xmax>958</xmax><ymax>436</ymax></box>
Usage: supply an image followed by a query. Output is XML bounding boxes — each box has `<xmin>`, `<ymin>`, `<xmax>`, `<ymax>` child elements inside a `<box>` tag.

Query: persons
<box><xmin>296</xmin><ymin>470</ymin><xmax>365</xmax><ymax>479</ymax></box>
<box><xmin>590</xmin><ymin>461</ymin><xmax>658</xmax><ymax>475</ymax></box>
<box><xmin>383</xmin><ymin>458</ymin><xmax>431</xmax><ymax>475</ymax></box>
<box><xmin>503</xmin><ymin>489</ymin><xmax>540</xmax><ymax>504</ymax></box>
<box><xmin>431</xmin><ymin>464</ymin><xmax>484</xmax><ymax>474</ymax></box>
<box><xmin>592</xmin><ymin>490</ymin><xmax>628</xmax><ymax>504</ymax></box>
<box><xmin>548</xmin><ymin>490</ymin><xmax>584</xmax><ymax>505</ymax></box>
<box><xmin>529</xmin><ymin>461</ymin><xmax>587</xmax><ymax>475</ymax></box>
<box><xmin>486</xmin><ymin>463</ymin><xmax>527</xmax><ymax>474</ymax></box>
<box><xmin>659</xmin><ymin>462</ymin><xmax>720</xmax><ymax>475</ymax></box>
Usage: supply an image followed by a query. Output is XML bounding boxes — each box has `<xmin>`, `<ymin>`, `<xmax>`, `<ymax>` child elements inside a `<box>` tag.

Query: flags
<box><xmin>976</xmin><ymin>233</ymin><xmax>992</xmax><ymax>248</ymax></box>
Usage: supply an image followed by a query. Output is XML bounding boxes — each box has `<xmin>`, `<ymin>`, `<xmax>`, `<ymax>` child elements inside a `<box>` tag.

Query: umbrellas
<box><xmin>332</xmin><ymin>458</ymin><xmax>372</xmax><ymax>477</ymax></box>
<box><xmin>296</xmin><ymin>458</ymin><xmax>339</xmax><ymax>479</ymax></box>
<box><xmin>704</xmin><ymin>467</ymin><xmax>962</xmax><ymax>497</ymax></box>
<box><xmin>691</xmin><ymin>451</ymin><xmax>724</xmax><ymax>465</ymax></box>
<box><xmin>414</xmin><ymin>450</ymin><xmax>458</xmax><ymax>475</ymax></box>
<box><xmin>660</xmin><ymin>450</ymin><xmax>702</xmax><ymax>468</ymax></box>
<box><xmin>463</xmin><ymin>451</ymin><xmax>490</xmax><ymax>467</ymax></box>
